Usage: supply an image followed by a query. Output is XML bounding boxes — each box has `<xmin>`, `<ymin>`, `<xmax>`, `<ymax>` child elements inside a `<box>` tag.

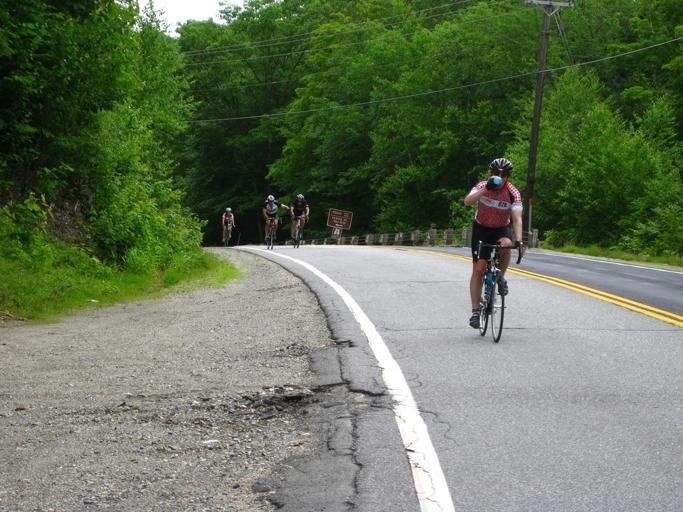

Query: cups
<box><xmin>493</xmin><ymin>177</ymin><xmax>504</xmax><ymax>187</ymax></box>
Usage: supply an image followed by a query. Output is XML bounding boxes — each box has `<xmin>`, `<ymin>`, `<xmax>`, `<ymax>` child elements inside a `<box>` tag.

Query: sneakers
<box><xmin>469</xmin><ymin>307</ymin><xmax>481</xmax><ymax>330</ymax></box>
<box><xmin>493</xmin><ymin>270</ymin><xmax>509</xmax><ymax>295</ymax></box>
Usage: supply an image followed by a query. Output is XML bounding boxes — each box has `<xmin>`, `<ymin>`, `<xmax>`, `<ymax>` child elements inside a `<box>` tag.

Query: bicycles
<box><xmin>293</xmin><ymin>214</ymin><xmax>309</xmax><ymax>248</ymax></box>
<box><xmin>266</xmin><ymin>216</ymin><xmax>283</xmax><ymax>249</ymax></box>
<box><xmin>224</xmin><ymin>221</ymin><xmax>235</xmax><ymax>247</ymax></box>
<box><xmin>474</xmin><ymin>239</ymin><xmax>522</xmax><ymax>342</ymax></box>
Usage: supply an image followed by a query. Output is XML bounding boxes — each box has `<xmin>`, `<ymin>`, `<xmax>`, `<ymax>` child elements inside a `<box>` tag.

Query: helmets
<box><xmin>226</xmin><ymin>207</ymin><xmax>232</xmax><ymax>212</ymax></box>
<box><xmin>297</xmin><ymin>194</ymin><xmax>305</xmax><ymax>201</ymax></box>
<box><xmin>268</xmin><ymin>195</ymin><xmax>275</xmax><ymax>201</ymax></box>
<box><xmin>490</xmin><ymin>159</ymin><xmax>513</xmax><ymax>175</ymax></box>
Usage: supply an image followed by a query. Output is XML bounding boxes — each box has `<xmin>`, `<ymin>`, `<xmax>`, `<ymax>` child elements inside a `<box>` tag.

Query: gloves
<box><xmin>485</xmin><ymin>177</ymin><xmax>497</xmax><ymax>191</ymax></box>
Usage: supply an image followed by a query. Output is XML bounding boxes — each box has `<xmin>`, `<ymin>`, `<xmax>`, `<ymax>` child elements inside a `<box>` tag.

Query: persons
<box><xmin>463</xmin><ymin>157</ymin><xmax>522</xmax><ymax>329</ymax></box>
<box><xmin>290</xmin><ymin>193</ymin><xmax>309</xmax><ymax>240</ymax></box>
<box><xmin>262</xmin><ymin>194</ymin><xmax>290</xmax><ymax>240</ymax></box>
<box><xmin>220</xmin><ymin>207</ymin><xmax>236</xmax><ymax>242</ymax></box>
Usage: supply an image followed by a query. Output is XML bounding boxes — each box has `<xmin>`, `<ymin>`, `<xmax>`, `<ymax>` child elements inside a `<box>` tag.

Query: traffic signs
<box><xmin>326</xmin><ymin>208</ymin><xmax>353</xmax><ymax>231</ymax></box>
